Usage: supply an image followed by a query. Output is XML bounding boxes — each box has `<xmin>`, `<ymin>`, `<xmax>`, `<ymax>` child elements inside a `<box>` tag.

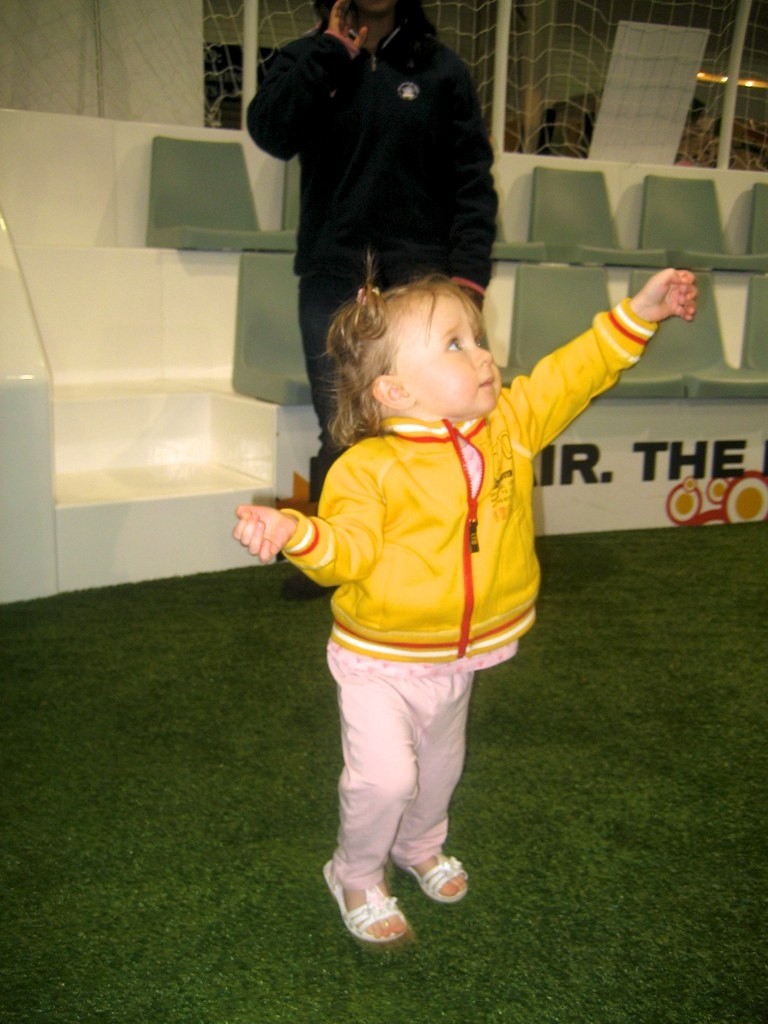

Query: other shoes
<box><xmin>282</xmin><ymin>572</ymin><xmax>336</xmax><ymax>601</ymax></box>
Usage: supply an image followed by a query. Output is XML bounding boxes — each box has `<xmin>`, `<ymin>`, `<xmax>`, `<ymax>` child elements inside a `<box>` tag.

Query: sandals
<box><xmin>323</xmin><ymin>859</ymin><xmax>407</xmax><ymax>942</ymax></box>
<box><xmin>392</xmin><ymin>852</ymin><xmax>468</xmax><ymax>903</ymax></box>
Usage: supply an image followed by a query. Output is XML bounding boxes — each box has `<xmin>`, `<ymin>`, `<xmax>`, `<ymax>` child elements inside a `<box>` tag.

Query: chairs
<box><xmin>1</xmin><ymin>108</ymin><xmax>768</xmax><ymax>603</ymax></box>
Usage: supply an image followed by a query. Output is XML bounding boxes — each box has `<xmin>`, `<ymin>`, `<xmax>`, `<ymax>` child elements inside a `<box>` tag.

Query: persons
<box><xmin>243</xmin><ymin>2</ymin><xmax>502</xmax><ymax>605</ymax></box>
<box><xmin>225</xmin><ymin>266</ymin><xmax>701</xmax><ymax>946</ymax></box>
